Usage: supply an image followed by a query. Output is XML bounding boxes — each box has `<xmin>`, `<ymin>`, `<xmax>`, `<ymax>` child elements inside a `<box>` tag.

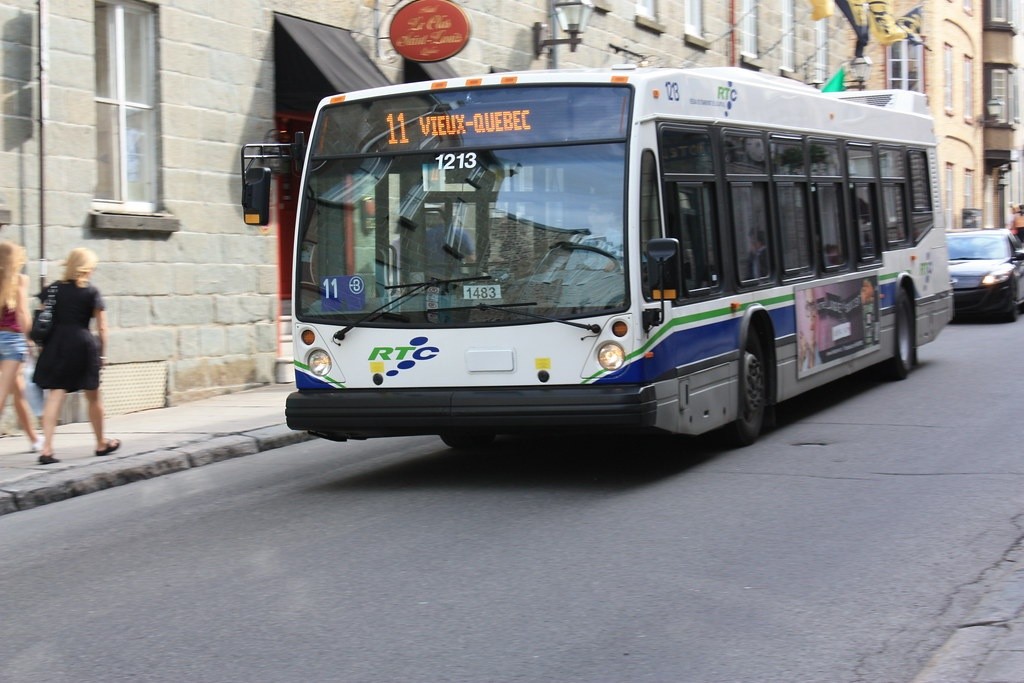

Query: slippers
<box><xmin>40</xmin><ymin>453</ymin><xmax>59</xmax><ymax>464</ymax></box>
<box><xmin>96</xmin><ymin>438</ymin><xmax>120</xmax><ymax>456</ymax></box>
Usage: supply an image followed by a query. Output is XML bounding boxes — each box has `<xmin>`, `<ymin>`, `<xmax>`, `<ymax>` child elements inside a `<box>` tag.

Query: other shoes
<box><xmin>32</xmin><ymin>436</ymin><xmax>46</xmax><ymax>452</ymax></box>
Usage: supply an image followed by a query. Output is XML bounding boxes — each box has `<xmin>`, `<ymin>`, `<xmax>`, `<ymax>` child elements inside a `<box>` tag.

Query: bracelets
<box><xmin>100</xmin><ymin>356</ymin><xmax>108</xmax><ymax>360</ymax></box>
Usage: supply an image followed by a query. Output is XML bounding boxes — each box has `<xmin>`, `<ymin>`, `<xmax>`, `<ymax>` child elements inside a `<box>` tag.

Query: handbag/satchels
<box><xmin>31</xmin><ymin>282</ymin><xmax>58</xmax><ymax>343</ymax></box>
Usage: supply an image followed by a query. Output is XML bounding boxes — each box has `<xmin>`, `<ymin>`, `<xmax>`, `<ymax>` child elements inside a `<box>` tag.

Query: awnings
<box><xmin>405</xmin><ymin>58</ymin><xmax>459</xmax><ymax>83</ymax></box>
<box><xmin>273</xmin><ymin>14</ymin><xmax>393</xmax><ymax>91</ymax></box>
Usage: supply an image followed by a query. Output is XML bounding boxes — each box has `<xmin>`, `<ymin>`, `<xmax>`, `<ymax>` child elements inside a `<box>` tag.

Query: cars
<box><xmin>945</xmin><ymin>227</ymin><xmax>1024</xmax><ymax>326</ymax></box>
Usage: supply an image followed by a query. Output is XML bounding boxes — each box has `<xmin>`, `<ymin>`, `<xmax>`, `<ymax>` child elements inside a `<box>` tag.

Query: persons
<box><xmin>390</xmin><ymin>213</ymin><xmax>476</xmax><ymax>295</ymax></box>
<box><xmin>747</xmin><ymin>228</ymin><xmax>768</xmax><ymax>279</ymax></box>
<box><xmin>799</xmin><ymin>288</ymin><xmax>822</xmax><ymax>372</ymax></box>
<box><xmin>30</xmin><ymin>246</ymin><xmax>121</xmax><ymax>463</ymax></box>
<box><xmin>565</xmin><ymin>198</ymin><xmax>647</xmax><ymax>274</ymax></box>
<box><xmin>1008</xmin><ymin>204</ymin><xmax>1024</xmax><ymax>243</ymax></box>
<box><xmin>0</xmin><ymin>241</ymin><xmax>45</xmax><ymax>453</ymax></box>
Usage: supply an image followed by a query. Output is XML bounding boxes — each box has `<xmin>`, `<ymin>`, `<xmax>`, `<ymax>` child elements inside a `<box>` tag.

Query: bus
<box><xmin>238</xmin><ymin>61</ymin><xmax>953</xmax><ymax>454</ymax></box>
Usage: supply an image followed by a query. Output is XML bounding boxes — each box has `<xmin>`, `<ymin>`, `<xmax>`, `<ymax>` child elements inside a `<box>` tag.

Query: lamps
<box><xmin>996</xmin><ymin>176</ymin><xmax>1009</xmax><ymax>192</ymax></box>
<box><xmin>532</xmin><ymin>0</ymin><xmax>593</xmax><ymax>60</ymax></box>
<box><xmin>975</xmin><ymin>95</ymin><xmax>1005</xmax><ymax>126</ymax></box>
<box><xmin>842</xmin><ymin>52</ymin><xmax>873</xmax><ymax>91</ymax></box>
